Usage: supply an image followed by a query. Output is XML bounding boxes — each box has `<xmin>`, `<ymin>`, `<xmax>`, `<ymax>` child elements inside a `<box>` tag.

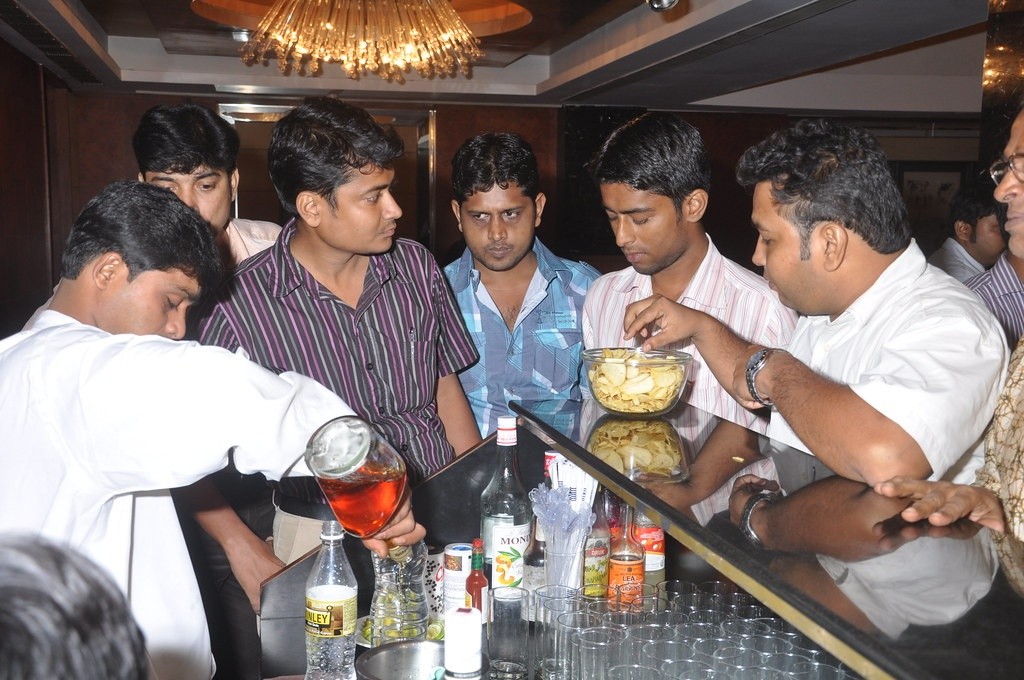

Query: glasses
<box><xmin>990</xmin><ymin>151</ymin><xmax>1024</xmax><ymax>187</ymax></box>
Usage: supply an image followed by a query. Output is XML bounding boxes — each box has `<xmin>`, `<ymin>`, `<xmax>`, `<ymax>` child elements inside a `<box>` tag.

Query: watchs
<box><xmin>738</xmin><ymin>495</ymin><xmax>770</xmax><ymax>550</ymax></box>
<box><xmin>745</xmin><ymin>347</ymin><xmax>787</xmax><ymax>407</ymax></box>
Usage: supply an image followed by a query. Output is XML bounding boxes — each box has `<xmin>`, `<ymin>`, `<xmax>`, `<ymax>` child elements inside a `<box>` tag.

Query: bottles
<box><xmin>478</xmin><ymin>415</ymin><xmax>667</xmax><ymax>633</ymax></box>
<box><xmin>466</xmin><ymin>537</ymin><xmax>489</xmax><ymax>628</ymax></box>
<box><xmin>304</xmin><ymin>416</ymin><xmax>413</xmax><ymax>564</ymax></box>
<box><xmin>303</xmin><ymin>521</ymin><xmax>358</xmax><ymax>680</ymax></box>
<box><xmin>370</xmin><ymin>540</ymin><xmax>429</xmax><ymax>650</ymax></box>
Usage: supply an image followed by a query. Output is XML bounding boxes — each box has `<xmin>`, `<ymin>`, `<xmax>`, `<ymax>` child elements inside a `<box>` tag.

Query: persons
<box><xmin>176</xmin><ymin>94</ymin><xmax>484</xmax><ymax>640</ymax></box>
<box><xmin>0</xmin><ymin>528</ymin><xmax>150</xmax><ymax>680</ymax></box>
<box><xmin>581</xmin><ymin>111</ymin><xmax>795</xmax><ymax>524</ymax></box>
<box><xmin>871</xmin><ymin>79</ymin><xmax>1024</xmax><ymax>538</ymax></box>
<box><xmin>0</xmin><ymin>180</ymin><xmax>426</xmax><ymax>680</ymax></box>
<box><xmin>621</xmin><ymin>118</ymin><xmax>1008</xmax><ymax>492</ymax></box>
<box><xmin>583</xmin><ymin>394</ymin><xmax>998</xmax><ymax>644</ymax></box>
<box><xmin>436</xmin><ymin>132</ymin><xmax>602</xmax><ymax>443</ymax></box>
<box><xmin>132</xmin><ymin>103</ymin><xmax>288</xmax><ymax>613</ymax></box>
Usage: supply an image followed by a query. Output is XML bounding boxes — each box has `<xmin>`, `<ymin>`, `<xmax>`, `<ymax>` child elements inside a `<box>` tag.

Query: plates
<box><xmin>355</xmin><ymin>612</ymin><xmax>445</xmax><ymax>649</ymax></box>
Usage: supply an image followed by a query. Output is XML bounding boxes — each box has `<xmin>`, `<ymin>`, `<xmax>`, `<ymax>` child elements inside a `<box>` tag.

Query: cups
<box><xmin>486</xmin><ymin>579</ymin><xmax>865</xmax><ymax>680</ymax></box>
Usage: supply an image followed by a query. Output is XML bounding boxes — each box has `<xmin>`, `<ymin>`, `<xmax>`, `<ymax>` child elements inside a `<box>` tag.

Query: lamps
<box><xmin>188</xmin><ymin>0</ymin><xmax>533</xmax><ymax>81</ymax></box>
<box><xmin>645</xmin><ymin>0</ymin><xmax>679</xmax><ymax>12</ymax></box>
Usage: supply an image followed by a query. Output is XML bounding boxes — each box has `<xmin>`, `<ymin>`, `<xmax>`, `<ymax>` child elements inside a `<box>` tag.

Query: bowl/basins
<box><xmin>354</xmin><ymin>640</ymin><xmax>489</xmax><ymax>680</ymax></box>
<box><xmin>583</xmin><ymin>414</ymin><xmax>689</xmax><ymax>485</ymax></box>
<box><xmin>581</xmin><ymin>347</ymin><xmax>694</xmax><ymax>420</ymax></box>
